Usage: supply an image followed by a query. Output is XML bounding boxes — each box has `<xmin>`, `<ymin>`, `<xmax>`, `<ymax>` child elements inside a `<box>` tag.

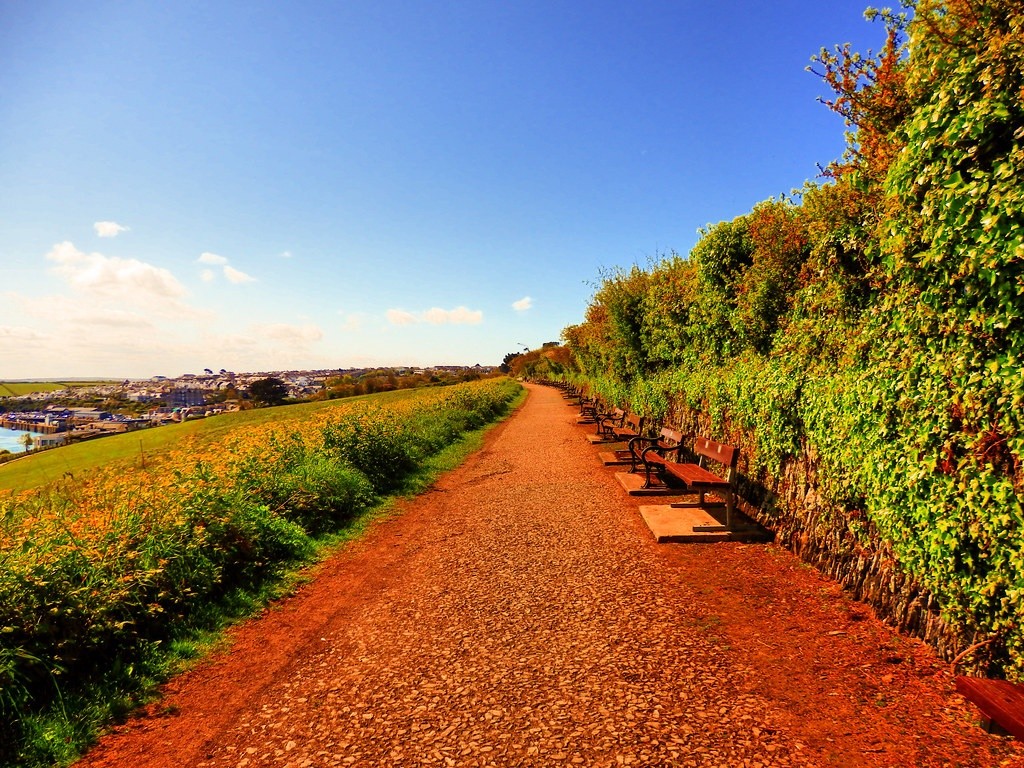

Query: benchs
<box><xmin>535</xmin><ymin>379</ymin><xmax>685</xmax><ymax>497</ymax></box>
<box><xmin>665</xmin><ymin>436</ymin><xmax>749</xmax><ymax>531</ymax></box>
